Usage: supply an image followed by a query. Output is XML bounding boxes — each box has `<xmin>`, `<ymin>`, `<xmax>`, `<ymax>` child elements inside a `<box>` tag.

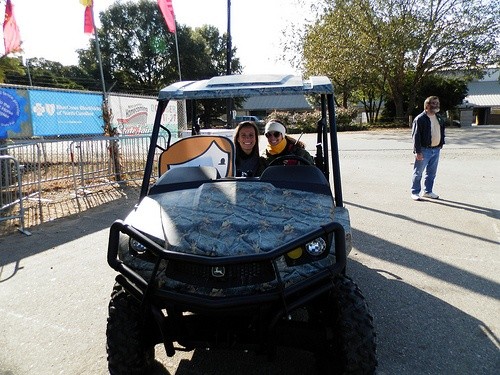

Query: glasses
<box><xmin>266</xmin><ymin>131</ymin><xmax>280</xmax><ymax>138</ymax></box>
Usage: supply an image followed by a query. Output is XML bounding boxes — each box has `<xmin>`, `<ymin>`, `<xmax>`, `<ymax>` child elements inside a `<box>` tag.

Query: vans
<box><xmin>236</xmin><ymin>115</ymin><xmax>260</xmax><ymax>126</ymax></box>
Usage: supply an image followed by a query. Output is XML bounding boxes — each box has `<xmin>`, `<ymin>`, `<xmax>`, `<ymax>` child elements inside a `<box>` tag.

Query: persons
<box><xmin>232</xmin><ymin>121</ymin><xmax>266</xmax><ymax>177</ymax></box>
<box><xmin>263</xmin><ymin>120</ymin><xmax>315</xmax><ymax>166</ymax></box>
<box><xmin>409</xmin><ymin>96</ymin><xmax>447</xmax><ymax>201</ymax></box>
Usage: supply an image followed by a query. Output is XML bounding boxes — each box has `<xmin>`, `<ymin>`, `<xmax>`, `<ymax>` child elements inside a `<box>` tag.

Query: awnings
<box><xmin>465</xmin><ymin>94</ymin><xmax>500</xmax><ymax>107</ymax></box>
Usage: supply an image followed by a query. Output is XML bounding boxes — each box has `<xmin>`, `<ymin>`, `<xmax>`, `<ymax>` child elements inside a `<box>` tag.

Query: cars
<box><xmin>210</xmin><ymin>119</ymin><xmax>228</xmax><ymax>127</ymax></box>
<box><xmin>106</xmin><ymin>74</ymin><xmax>378</xmax><ymax>375</ymax></box>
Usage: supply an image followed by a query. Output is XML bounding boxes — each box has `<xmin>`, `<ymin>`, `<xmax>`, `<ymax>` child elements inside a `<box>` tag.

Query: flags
<box><xmin>156</xmin><ymin>0</ymin><xmax>176</xmax><ymax>33</ymax></box>
<box><xmin>81</xmin><ymin>0</ymin><xmax>93</xmax><ymax>34</ymax></box>
<box><xmin>2</xmin><ymin>0</ymin><xmax>22</xmax><ymax>54</ymax></box>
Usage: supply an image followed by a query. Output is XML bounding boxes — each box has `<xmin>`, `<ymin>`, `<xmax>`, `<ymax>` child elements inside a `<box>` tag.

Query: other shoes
<box><xmin>422</xmin><ymin>192</ymin><xmax>439</xmax><ymax>199</ymax></box>
<box><xmin>410</xmin><ymin>194</ymin><xmax>422</xmax><ymax>200</ymax></box>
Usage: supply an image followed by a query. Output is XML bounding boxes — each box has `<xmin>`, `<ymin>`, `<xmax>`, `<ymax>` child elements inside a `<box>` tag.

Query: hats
<box><xmin>265</xmin><ymin>120</ymin><xmax>285</xmax><ymax>138</ymax></box>
